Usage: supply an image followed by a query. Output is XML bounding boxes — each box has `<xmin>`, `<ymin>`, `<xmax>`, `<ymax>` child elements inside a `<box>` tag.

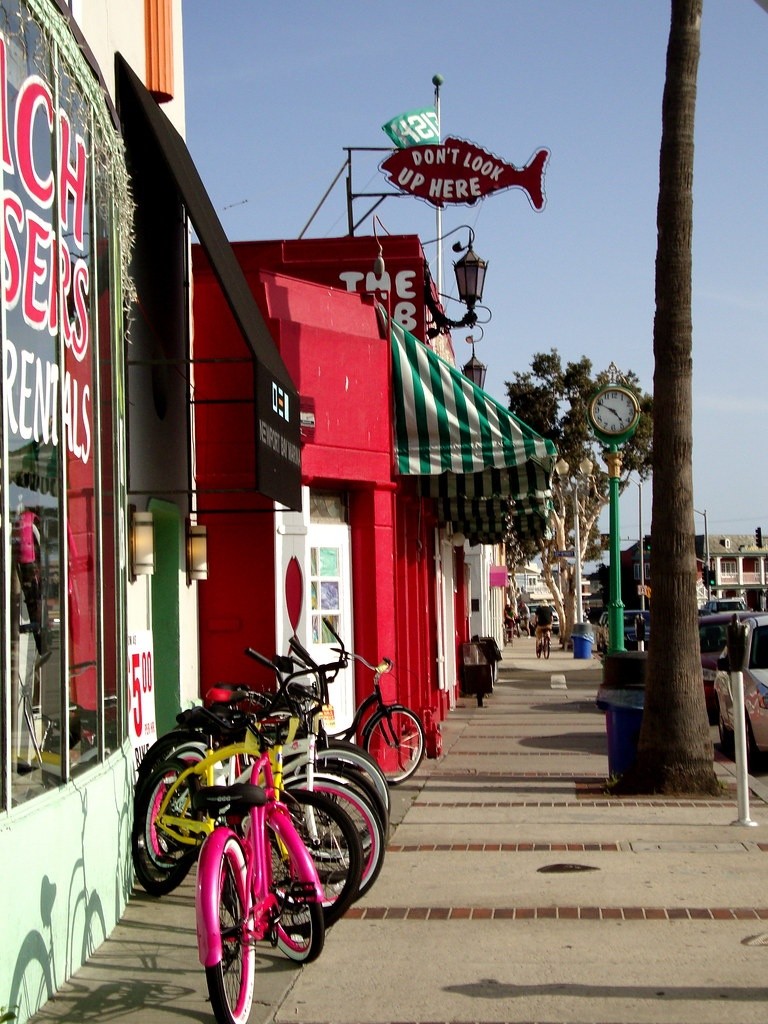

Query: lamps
<box><xmin>440</xmin><ymin>325</ymin><xmax>488</xmax><ymax>390</ymax></box>
<box><xmin>443</xmin><ymin>532</ymin><xmax>465</xmax><ymax>547</ymax></box>
<box><xmin>184</xmin><ymin>517</ymin><xmax>208</xmax><ymax>586</ymax></box>
<box><xmin>373</xmin><ymin>214</ymin><xmax>390</xmax><ymax>281</ymax></box>
<box><xmin>425</xmin><ymin>230</ymin><xmax>489</xmax><ymax>339</ymax></box>
<box><xmin>127</xmin><ymin>503</ymin><xmax>153</xmax><ymax>584</ymax></box>
<box><xmin>422</xmin><ymin>225</ymin><xmax>475</xmax><ymax>252</ymax></box>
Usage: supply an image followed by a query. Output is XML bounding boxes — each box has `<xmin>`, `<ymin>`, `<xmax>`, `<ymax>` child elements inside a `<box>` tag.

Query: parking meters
<box><xmin>716</xmin><ymin>613</ymin><xmax>758</xmax><ymax>827</ymax></box>
<box><xmin>634</xmin><ymin>613</ymin><xmax>645</xmax><ymax>652</ymax></box>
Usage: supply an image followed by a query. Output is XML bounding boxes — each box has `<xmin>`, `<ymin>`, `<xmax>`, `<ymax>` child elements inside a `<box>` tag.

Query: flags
<box><xmin>381</xmin><ymin>106</ymin><xmax>439</xmax><ymax>150</ymax></box>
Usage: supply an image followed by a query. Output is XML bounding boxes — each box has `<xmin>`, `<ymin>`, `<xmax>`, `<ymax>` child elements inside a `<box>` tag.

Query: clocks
<box><xmin>591</xmin><ymin>388</ymin><xmax>639</xmax><ymax>435</ymax></box>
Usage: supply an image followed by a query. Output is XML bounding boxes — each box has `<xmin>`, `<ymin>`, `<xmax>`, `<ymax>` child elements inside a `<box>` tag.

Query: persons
<box><xmin>503</xmin><ymin>602</ymin><xmax>532</xmax><ymax>639</ymax></box>
<box><xmin>534</xmin><ymin>600</ymin><xmax>553</xmax><ymax>647</ymax></box>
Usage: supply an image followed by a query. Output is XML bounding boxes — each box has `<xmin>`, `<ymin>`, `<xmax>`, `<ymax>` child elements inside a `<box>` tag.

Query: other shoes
<box><xmin>537</xmin><ymin>643</ymin><xmax>541</xmax><ymax>652</ymax></box>
<box><xmin>548</xmin><ymin>637</ymin><xmax>551</xmax><ymax>644</ymax></box>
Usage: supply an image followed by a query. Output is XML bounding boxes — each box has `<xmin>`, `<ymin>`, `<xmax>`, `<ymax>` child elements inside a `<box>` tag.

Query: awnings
<box><xmin>379</xmin><ymin>303</ymin><xmax>558</xmax><ymax>546</ymax></box>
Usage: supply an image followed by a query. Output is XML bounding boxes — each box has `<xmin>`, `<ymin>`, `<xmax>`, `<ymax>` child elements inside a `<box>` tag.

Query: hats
<box><xmin>539</xmin><ymin>599</ymin><xmax>549</xmax><ymax>608</ymax></box>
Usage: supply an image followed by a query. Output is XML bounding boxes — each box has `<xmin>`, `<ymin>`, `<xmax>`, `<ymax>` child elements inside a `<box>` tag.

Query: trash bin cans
<box><xmin>573</xmin><ymin>623</ymin><xmax>592</xmax><ymax>659</ymax></box>
<box><xmin>597</xmin><ymin>652</ymin><xmax>649</xmax><ymax>780</ymax></box>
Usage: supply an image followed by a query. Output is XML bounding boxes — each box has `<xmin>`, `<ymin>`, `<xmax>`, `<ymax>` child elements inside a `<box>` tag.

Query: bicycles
<box><xmin>132</xmin><ymin>618</ymin><xmax>426</xmax><ymax>1024</ymax></box>
<box><xmin>532</xmin><ymin>622</ymin><xmax>551</xmax><ymax>659</ymax></box>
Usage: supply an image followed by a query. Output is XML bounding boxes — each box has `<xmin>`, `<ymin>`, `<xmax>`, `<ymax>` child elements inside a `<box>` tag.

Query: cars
<box><xmin>526</xmin><ymin>603</ymin><xmax>559</xmax><ymax>636</ymax></box>
<box><xmin>712</xmin><ymin>616</ymin><xmax>768</xmax><ymax>771</ymax></box>
<box><xmin>596</xmin><ymin>609</ymin><xmax>650</xmax><ymax>654</ymax></box>
<box><xmin>699</xmin><ymin>611</ymin><xmax>768</xmax><ymax>712</ymax></box>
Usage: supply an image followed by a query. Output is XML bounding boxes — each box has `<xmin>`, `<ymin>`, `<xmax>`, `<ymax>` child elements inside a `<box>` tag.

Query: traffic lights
<box><xmin>701</xmin><ymin>565</ymin><xmax>709</xmax><ymax>589</ymax></box>
<box><xmin>755</xmin><ymin>527</ymin><xmax>762</xmax><ymax>548</ymax></box>
<box><xmin>709</xmin><ymin>570</ymin><xmax>718</xmax><ymax>586</ymax></box>
